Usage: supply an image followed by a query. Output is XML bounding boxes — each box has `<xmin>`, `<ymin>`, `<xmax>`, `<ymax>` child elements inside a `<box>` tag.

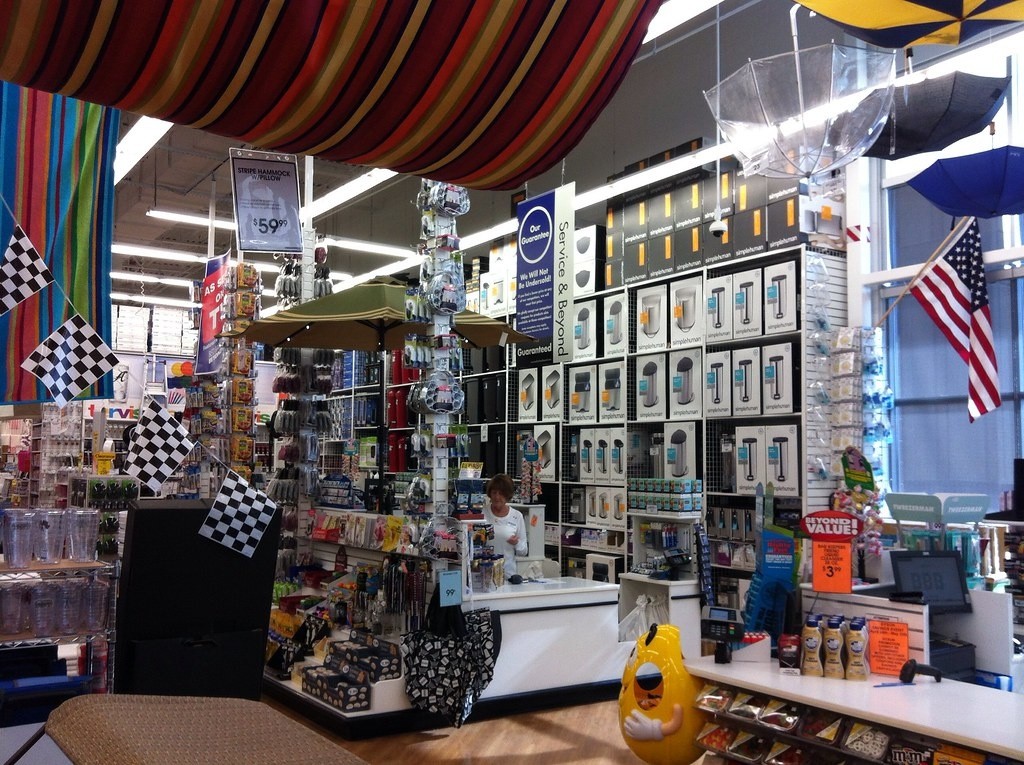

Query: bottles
<box><xmin>800</xmin><ymin>614</ymin><xmax>871</xmax><ymax>681</ymax></box>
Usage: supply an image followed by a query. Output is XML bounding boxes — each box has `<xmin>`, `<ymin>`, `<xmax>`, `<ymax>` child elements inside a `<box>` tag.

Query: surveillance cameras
<box><xmin>709</xmin><ymin>221</ymin><xmax>727</xmax><ymax>237</ymax></box>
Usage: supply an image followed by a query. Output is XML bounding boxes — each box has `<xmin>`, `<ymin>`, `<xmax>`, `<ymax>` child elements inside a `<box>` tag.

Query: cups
<box><xmin>0</xmin><ymin>508</ymin><xmax>110</xmax><ymax>636</ymax></box>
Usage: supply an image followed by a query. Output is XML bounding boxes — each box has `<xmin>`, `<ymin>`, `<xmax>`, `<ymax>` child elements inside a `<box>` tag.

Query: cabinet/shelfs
<box><xmin>26</xmin><ymin>401</ymin><xmax>85</xmax><ymax>511</ymax></box>
<box><xmin>315</xmin><ymin>146</ymin><xmax>846</xmax><ymax>585</ymax></box>
<box><xmin>85</xmin><ymin>417</ymin><xmax>139</xmax><ymax>479</ymax></box>
<box><xmin>0</xmin><ymin>556</ymin><xmax>116</xmax><ymax>727</ymax></box>
<box><xmin>253</xmin><ymin>422</ymin><xmax>274</xmax><ymax>461</ymax></box>
<box><xmin>688</xmin><ymin>651</ymin><xmax>1024</xmax><ymax>765</ymax></box>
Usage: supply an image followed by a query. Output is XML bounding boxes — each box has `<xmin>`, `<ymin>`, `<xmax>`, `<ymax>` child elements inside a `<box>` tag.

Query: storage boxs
<box><xmin>320</xmin><ymin>146</ymin><xmax>800</xmax><ymax>586</ymax></box>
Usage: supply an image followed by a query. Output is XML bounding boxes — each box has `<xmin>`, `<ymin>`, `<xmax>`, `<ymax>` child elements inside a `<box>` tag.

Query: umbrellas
<box><xmin>700</xmin><ymin>4</ymin><xmax>898</xmax><ymax>179</ymax></box>
<box><xmin>906</xmin><ymin>122</ymin><xmax>1024</xmax><ymax>217</ymax></box>
<box><xmin>792</xmin><ymin>0</ymin><xmax>1024</xmax><ymax>49</ymax></box>
<box><xmin>827</xmin><ymin>51</ymin><xmax>1012</xmax><ymax>161</ymax></box>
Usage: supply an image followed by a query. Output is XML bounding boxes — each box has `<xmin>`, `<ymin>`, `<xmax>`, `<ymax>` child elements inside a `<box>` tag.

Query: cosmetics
<box><xmin>802</xmin><ymin>612</ymin><xmax>871</xmax><ymax>680</ymax></box>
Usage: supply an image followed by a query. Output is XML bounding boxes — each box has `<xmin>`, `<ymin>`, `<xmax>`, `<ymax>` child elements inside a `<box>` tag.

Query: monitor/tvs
<box><xmin>890</xmin><ymin>550</ymin><xmax>973</xmax><ymax>614</ymax></box>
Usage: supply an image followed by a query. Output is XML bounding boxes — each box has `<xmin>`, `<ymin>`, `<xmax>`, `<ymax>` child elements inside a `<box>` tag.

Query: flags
<box><xmin>199</xmin><ymin>468</ymin><xmax>277</xmax><ymax>558</ymax></box>
<box><xmin>0</xmin><ymin>224</ymin><xmax>56</xmax><ymax>315</ymax></box>
<box><xmin>910</xmin><ymin>216</ymin><xmax>1006</xmax><ymax>424</ymax></box>
<box><xmin>20</xmin><ymin>313</ymin><xmax>122</xmax><ymax>408</ymax></box>
<box><xmin>122</xmin><ymin>398</ymin><xmax>198</xmax><ymax>493</ymax></box>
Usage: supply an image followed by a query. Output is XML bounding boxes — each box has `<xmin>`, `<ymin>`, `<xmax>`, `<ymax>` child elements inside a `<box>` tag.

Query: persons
<box><xmin>477</xmin><ymin>472</ymin><xmax>529</xmax><ymax>579</ymax></box>
